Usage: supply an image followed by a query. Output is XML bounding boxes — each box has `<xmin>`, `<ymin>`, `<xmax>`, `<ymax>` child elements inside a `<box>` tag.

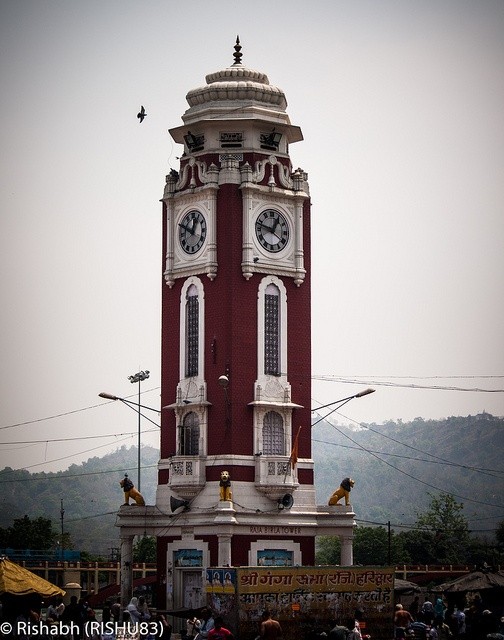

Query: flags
<box><xmin>290</xmin><ymin>425</ymin><xmax>301</xmax><ymax>470</ymax></box>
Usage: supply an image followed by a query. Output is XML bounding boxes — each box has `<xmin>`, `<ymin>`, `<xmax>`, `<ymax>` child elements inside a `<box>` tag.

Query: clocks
<box><xmin>176</xmin><ymin>209</ymin><xmax>206</xmax><ymax>255</ymax></box>
<box><xmin>254</xmin><ymin>208</ymin><xmax>289</xmax><ymax>253</ymax></box>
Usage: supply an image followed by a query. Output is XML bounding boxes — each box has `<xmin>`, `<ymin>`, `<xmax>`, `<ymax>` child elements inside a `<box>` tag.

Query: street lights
<box><xmin>128</xmin><ymin>369</ymin><xmax>150</xmax><ymax>492</ymax></box>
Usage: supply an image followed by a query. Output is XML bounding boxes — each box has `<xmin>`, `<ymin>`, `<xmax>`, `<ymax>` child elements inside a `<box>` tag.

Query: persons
<box><xmin>0</xmin><ymin>596</ymin><xmax>173</xmax><ymax>640</ymax></box>
<box><xmin>318</xmin><ymin>611</ymin><xmax>371</xmax><ymax>640</ymax></box>
<box><xmin>189</xmin><ymin>608</ymin><xmax>214</xmax><ymax>640</ymax></box>
<box><xmin>205</xmin><ymin>616</ymin><xmax>235</xmax><ymax>640</ymax></box>
<box><xmin>185</xmin><ymin>609</ymin><xmax>201</xmax><ymax>640</ymax></box>
<box><xmin>393</xmin><ymin>593</ymin><xmax>504</xmax><ymax>640</ymax></box>
<box><xmin>253</xmin><ymin>611</ymin><xmax>283</xmax><ymax>640</ymax></box>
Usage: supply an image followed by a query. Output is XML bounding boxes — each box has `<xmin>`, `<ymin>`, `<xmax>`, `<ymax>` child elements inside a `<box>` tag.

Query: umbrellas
<box><xmin>0</xmin><ymin>553</ymin><xmax>66</xmax><ymax>597</ymax></box>
